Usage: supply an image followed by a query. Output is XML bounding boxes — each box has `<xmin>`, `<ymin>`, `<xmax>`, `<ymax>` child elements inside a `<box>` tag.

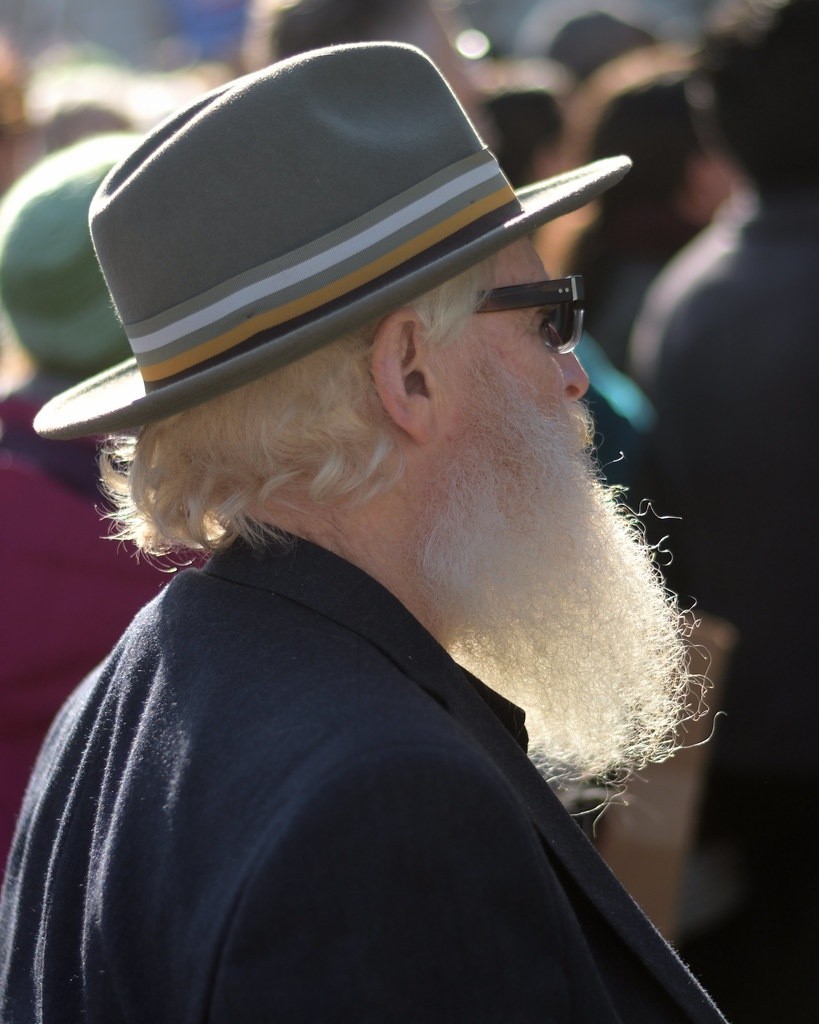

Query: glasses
<box><xmin>473</xmin><ymin>274</ymin><xmax>584</xmax><ymax>356</ymax></box>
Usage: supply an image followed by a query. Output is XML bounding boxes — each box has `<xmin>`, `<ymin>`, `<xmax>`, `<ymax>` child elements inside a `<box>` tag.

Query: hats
<box><xmin>34</xmin><ymin>42</ymin><xmax>630</xmax><ymax>442</ymax></box>
<box><xmin>1</xmin><ymin>131</ymin><xmax>134</xmax><ymax>365</ymax></box>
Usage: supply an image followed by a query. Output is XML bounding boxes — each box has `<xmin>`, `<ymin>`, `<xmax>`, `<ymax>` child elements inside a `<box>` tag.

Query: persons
<box><xmin>0</xmin><ymin>136</ymin><xmax>216</xmax><ymax>899</ymax></box>
<box><xmin>622</xmin><ymin>4</ymin><xmax>818</xmax><ymax>1024</ymax></box>
<box><xmin>532</xmin><ymin>47</ymin><xmax>741</xmax><ymax>496</ymax></box>
<box><xmin>230</xmin><ymin>0</ymin><xmax>645</xmax><ymax>165</ymax></box>
<box><xmin>1</xmin><ymin>39</ymin><xmax>732</xmax><ymax>1023</ymax></box>
